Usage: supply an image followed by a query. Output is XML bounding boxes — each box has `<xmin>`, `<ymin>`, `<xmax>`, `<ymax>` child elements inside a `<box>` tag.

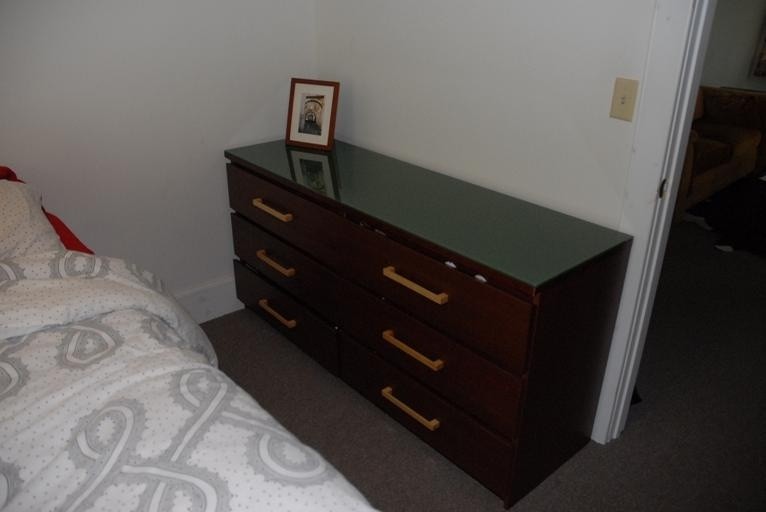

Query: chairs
<box><xmin>675</xmin><ymin>86</ymin><xmax>761</xmax><ymax>224</ymax></box>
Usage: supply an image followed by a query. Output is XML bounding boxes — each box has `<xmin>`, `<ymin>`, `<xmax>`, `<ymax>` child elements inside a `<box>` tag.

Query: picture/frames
<box><xmin>283</xmin><ymin>78</ymin><xmax>342</xmax><ymax>151</ymax></box>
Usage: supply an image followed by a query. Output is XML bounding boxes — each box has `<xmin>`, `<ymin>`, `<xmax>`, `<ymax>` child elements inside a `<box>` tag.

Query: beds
<box><xmin>0</xmin><ymin>162</ymin><xmax>381</xmax><ymax>510</ymax></box>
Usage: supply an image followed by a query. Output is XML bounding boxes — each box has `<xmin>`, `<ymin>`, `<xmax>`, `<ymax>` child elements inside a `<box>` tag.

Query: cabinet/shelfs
<box><xmin>223</xmin><ymin>157</ymin><xmax>634</xmax><ymax>511</ymax></box>
